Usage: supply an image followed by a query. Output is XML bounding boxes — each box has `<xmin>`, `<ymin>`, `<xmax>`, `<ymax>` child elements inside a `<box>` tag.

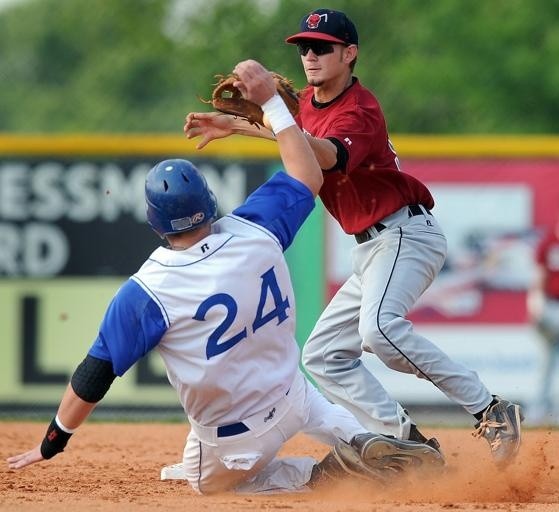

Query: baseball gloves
<box><xmin>211</xmin><ymin>72</ymin><xmax>302</xmax><ymax>128</ymax></box>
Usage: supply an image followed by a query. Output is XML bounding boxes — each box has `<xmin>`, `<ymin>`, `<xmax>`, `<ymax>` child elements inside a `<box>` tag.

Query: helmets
<box><xmin>143</xmin><ymin>158</ymin><xmax>218</xmax><ymax>240</ymax></box>
<box><xmin>283</xmin><ymin>7</ymin><xmax>360</xmax><ymax>60</ymax></box>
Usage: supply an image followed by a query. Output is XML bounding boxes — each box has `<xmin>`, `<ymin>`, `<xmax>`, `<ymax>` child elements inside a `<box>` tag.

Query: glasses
<box><xmin>296</xmin><ymin>42</ymin><xmax>336</xmax><ymax>57</ymax></box>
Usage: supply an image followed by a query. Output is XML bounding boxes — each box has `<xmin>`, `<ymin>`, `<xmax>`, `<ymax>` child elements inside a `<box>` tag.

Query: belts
<box><xmin>355</xmin><ymin>204</ymin><xmax>432</xmax><ymax>245</ymax></box>
<box><xmin>217</xmin><ymin>421</ymin><xmax>250</xmax><ymax>439</ymax></box>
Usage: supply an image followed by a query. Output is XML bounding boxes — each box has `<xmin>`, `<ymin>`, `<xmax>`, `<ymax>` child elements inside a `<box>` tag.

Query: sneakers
<box><xmin>470</xmin><ymin>394</ymin><xmax>526</xmax><ymax>473</ymax></box>
<box><xmin>306</xmin><ymin>422</ymin><xmax>448</xmax><ymax>496</ymax></box>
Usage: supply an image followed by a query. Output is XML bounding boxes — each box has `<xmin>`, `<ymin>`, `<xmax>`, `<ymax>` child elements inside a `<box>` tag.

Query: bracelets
<box><xmin>39</xmin><ymin>415</ymin><xmax>76</xmax><ymax>462</ymax></box>
<box><xmin>258</xmin><ymin>94</ymin><xmax>297</xmax><ymax>135</ymax></box>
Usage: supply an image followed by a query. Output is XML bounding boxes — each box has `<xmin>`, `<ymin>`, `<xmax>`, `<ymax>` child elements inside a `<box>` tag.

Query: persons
<box><xmin>522</xmin><ymin>220</ymin><xmax>559</xmax><ymax>427</ymax></box>
<box><xmin>183</xmin><ymin>6</ymin><xmax>526</xmax><ymax>474</ymax></box>
<box><xmin>10</xmin><ymin>57</ymin><xmax>445</xmax><ymax>499</ymax></box>
